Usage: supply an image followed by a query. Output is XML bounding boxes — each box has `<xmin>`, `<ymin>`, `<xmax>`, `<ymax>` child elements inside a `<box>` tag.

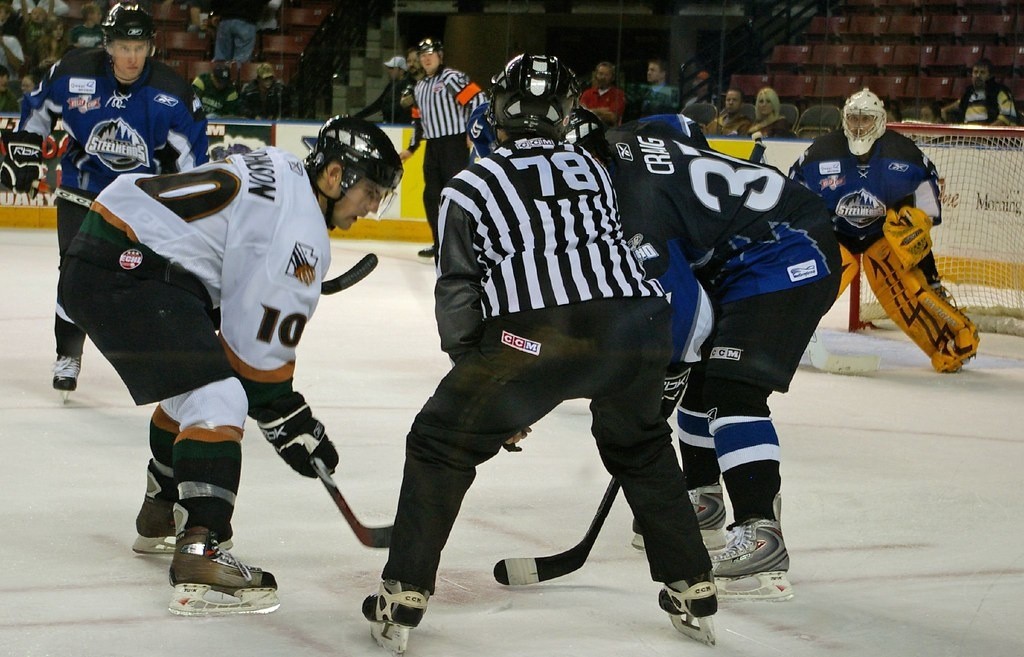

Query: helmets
<box><xmin>418</xmin><ymin>38</ymin><xmax>444</xmax><ymax>52</ymax></box>
<box><xmin>840</xmin><ymin>87</ymin><xmax>888</xmax><ymax>155</ymax></box>
<box><xmin>561</xmin><ymin>106</ymin><xmax>610</xmax><ymax>149</ymax></box>
<box><xmin>490</xmin><ymin>54</ymin><xmax>580</xmax><ymax>141</ymax></box>
<box><xmin>307</xmin><ymin>115</ymin><xmax>404</xmax><ymax>187</ymax></box>
<box><xmin>102</xmin><ymin>3</ymin><xmax>157</xmax><ymax>40</ymax></box>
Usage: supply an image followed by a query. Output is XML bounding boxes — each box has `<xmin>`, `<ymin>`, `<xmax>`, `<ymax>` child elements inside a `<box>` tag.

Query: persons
<box><xmin>0</xmin><ymin>0</ymin><xmax>69</xmax><ymax>115</ymax></box>
<box><xmin>188</xmin><ymin>0</ymin><xmax>280</xmax><ymax>63</ymax></box>
<box><xmin>190</xmin><ymin>60</ymin><xmax>239</xmax><ymax>117</ymax></box>
<box><xmin>353</xmin><ymin>45</ymin><xmax>425</xmax><ymax>124</ymax></box>
<box><xmin>750</xmin><ymin>86</ymin><xmax>797</xmax><ymax>138</ymax></box>
<box><xmin>705</xmin><ymin>89</ymin><xmax>750</xmax><ymax>138</ymax></box>
<box><xmin>67</xmin><ymin>2</ymin><xmax>103</xmax><ymax>49</ymax></box>
<box><xmin>363</xmin><ymin>52</ymin><xmax>718</xmax><ymax>627</ymax></box>
<box><xmin>0</xmin><ymin>5</ymin><xmax>212</xmax><ymax>389</ymax></box>
<box><xmin>942</xmin><ymin>59</ymin><xmax>1018</xmax><ymax>126</ymax></box>
<box><xmin>60</xmin><ymin>116</ymin><xmax>403</xmax><ymax>596</ymax></box>
<box><xmin>786</xmin><ymin>91</ymin><xmax>979</xmax><ymax>373</ymax></box>
<box><xmin>238</xmin><ymin>64</ymin><xmax>292</xmax><ymax>121</ymax></box>
<box><xmin>570</xmin><ymin>110</ymin><xmax>843</xmax><ymax>578</ymax></box>
<box><xmin>677</xmin><ymin>70</ymin><xmax>717</xmax><ymax>115</ymax></box>
<box><xmin>581</xmin><ymin>63</ymin><xmax>626</xmax><ymax>127</ymax></box>
<box><xmin>399</xmin><ymin>43</ymin><xmax>495</xmax><ymax>260</ymax></box>
<box><xmin>638</xmin><ymin>56</ymin><xmax>681</xmax><ymax>117</ymax></box>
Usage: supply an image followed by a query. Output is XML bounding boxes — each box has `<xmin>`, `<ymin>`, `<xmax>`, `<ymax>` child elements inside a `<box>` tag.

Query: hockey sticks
<box><xmin>493</xmin><ymin>136</ymin><xmax>768</xmax><ymax>587</ymax></box>
<box><xmin>310</xmin><ymin>452</ymin><xmax>394</xmax><ymax>549</ymax></box>
<box><xmin>29</xmin><ymin>180</ymin><xmax>379</xmax><ymax>296</ymax></box>
<box><xmin>749</xmin><ymin>130</ymin><xmax>887</xmax><ymax>374</ymax></box>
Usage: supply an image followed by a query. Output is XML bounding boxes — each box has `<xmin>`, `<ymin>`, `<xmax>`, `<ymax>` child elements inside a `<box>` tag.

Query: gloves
<box><xmin>252</xmin><ymin>393</ymin><xmax>338</xmax><ymax>477</ymax></box>
<box><xmin>0</xmin><ymin>129</ymin><xmax>45</xmax><ymax>201</ymax></box>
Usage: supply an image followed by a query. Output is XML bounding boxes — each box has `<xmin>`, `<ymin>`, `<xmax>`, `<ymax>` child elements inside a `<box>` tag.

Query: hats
<box><xmin>384</xmin><ymin>56</ymin><xmax>408</xmax><ymax>72</ymax></box>
<box><xmin>212</xmin><ymin>66</ymin><xmax>232</xmax><ymax>82</ymax></box>
<box><xmin>256</xmin><ymin>64</ymin><xmax>275</xmax><ymax>78</ymax></box>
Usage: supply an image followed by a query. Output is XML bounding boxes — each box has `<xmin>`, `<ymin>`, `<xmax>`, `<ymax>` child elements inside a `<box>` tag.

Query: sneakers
<box><xmin>417</xmin><ymin>245</ymin><xmax>436</xmax><ymax>265</ymax></box>
<box><xmin>658</xmin><ymin>571</ymin><xmax>718</xmax><ymax>648</ymax></box>
<box><xmin>169</xmin><ymin>504</ymin><xmax>281</xmax><ymax>617</ymax></box>
<box><xmin>53</xmin><ymin>355</ymin><xmax>81</xmax><ymax>404</ymax></box>
<box><xmin>632</xmin><ymin>477</ymin><xmax>726</xmax><ymax>550</ymax></box>
<box><xmin>361</xmin><ymin>580</ymin><xmax>429</xmax><ymax>656</ymax></box>
<box><xmin>713</xmin><ymin>491</ymin><xmax>796</xmax><ymax>601</ymax></box>
<box><xmin>133</xmin><ymin>457</ymin><xmax>234</xmax><ymax>557</ymax></box>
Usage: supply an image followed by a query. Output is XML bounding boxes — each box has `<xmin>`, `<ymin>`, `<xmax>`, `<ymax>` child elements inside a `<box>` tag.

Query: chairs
<box><xmin>154</xmin><ymin>0</ymin><xmax>345</xmax><ymax>121</ymax></box>
<box><xmin>717</xmin><ymin>0</ymin><xmax>1024</xmax><ymax>135</ymax></box>
